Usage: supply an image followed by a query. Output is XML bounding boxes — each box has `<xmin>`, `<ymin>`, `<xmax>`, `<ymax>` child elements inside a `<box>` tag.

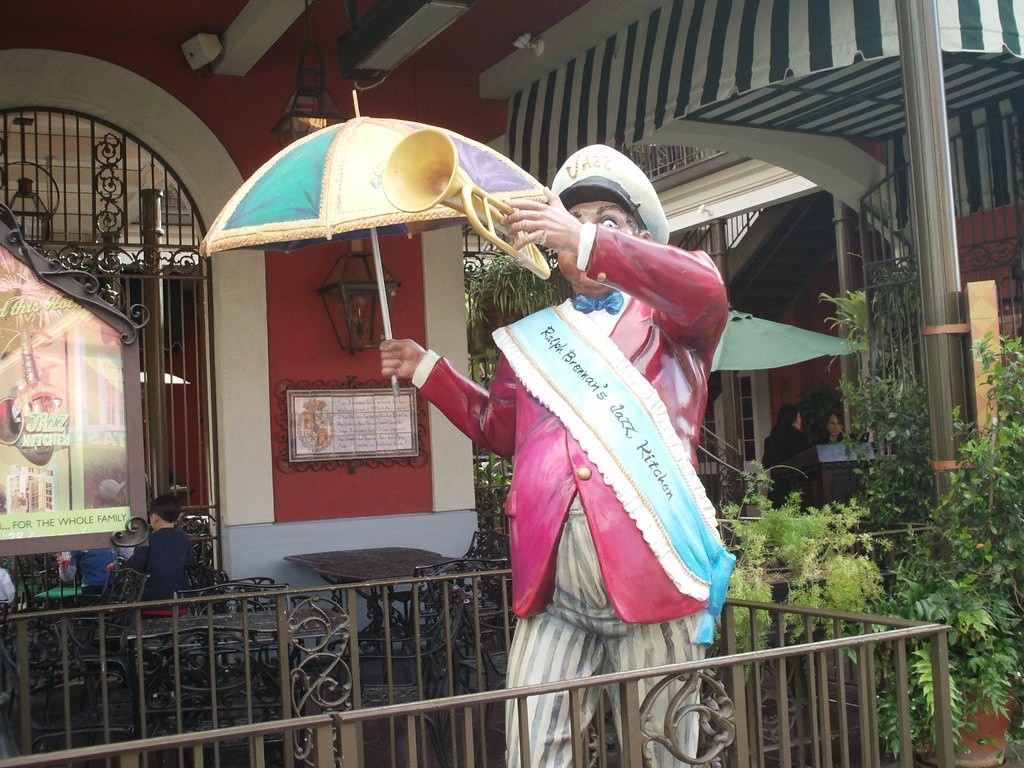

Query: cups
<box><xmin>62</xmin><ymin>552</ymin><xmax>71</xmax><ymax>560</ymax></box>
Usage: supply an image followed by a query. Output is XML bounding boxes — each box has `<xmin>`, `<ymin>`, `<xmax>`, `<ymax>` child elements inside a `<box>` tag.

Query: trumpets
<box><xmin>382</xmin><ymin>128</ymin><xmax>551</xmax><ymax>280</ymax></box>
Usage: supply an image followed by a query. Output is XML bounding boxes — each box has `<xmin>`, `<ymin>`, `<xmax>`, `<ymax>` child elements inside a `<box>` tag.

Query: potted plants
<box><xmin>848</xmin><ymin>526</ymin><xmax>1024</xmax><ymax>767</ymax></box>
<box><xmin>465</xmin><ymin>249</ymin><xmax>563</xmax><ymax>347</ymax></box>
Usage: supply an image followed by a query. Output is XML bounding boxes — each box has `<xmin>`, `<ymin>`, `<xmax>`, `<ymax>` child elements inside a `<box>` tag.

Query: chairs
<box><xmin>0</xmin><ymin>526</ymin><xmax>519</xmax><ymax>767</ymax></box>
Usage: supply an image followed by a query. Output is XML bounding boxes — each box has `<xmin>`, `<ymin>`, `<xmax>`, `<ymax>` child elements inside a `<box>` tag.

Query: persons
<box><xmin>126</xmin><ymin>495</ymin><xmax>192</xmax><ymax>619</ymax></box>
<box><xmin>762</xmin><ymin>404</ymin><xmax>815</xmax><ymax>501</ymax></box>
<box><xmin>0</xmin><ymin>568</ymin><xmax>15</xmax><ymax>616</ymax></box>
<box><xmin>56</xmin><ymin>547</ymin><xmax>134</xmax><ymax>628</ymax></box>
<box><xmin>376</xmin><ymin>144</ymin><xmax>731</xmax><ymax>768</ymax></box>
<box><xmin>813</xmin><ymin>412</ymin><xmax>844</xmax><ymax>445</ymax></box>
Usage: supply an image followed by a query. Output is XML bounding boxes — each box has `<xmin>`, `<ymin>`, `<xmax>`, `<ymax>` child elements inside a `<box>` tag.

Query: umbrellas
<box><xmin>198</xmin><ymin>89</ymin><xmax>550</xmax><ymax>398</ymax></box>
<box><xmin>711</xmin><ymin>311</ymin><xmax>865</xmax><ymax>371</ymax></box>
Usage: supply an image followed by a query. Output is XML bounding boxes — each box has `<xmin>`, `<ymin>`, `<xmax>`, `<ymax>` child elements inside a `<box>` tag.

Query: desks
<box><xmin>313</xmin><ymin>555</ymin><xmax>459</xmax><ymax>684</ymax></box>
<box><xmin>282</xmin><ymin>547</ymin><xmax>441</xmax><ymax>585</ymax></box>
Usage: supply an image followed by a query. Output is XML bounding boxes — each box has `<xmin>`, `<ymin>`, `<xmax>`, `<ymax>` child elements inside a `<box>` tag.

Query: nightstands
<box><xmin>127</xmin><ymin>606</ymin><xmax>350</xmax><ymax>767</ymax></box>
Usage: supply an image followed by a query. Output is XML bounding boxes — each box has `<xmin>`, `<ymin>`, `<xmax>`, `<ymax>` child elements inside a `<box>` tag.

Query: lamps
<box><xmin>318</xmin><ymin>239</ymin><xmax>402</xmax><ymax>357</ymax></box>
<box><xmin>9</xmin><ymin>117</ymin><xmax>50</xmax><ymax>249</ymax></box>
<box><xmin>513</xmin><ymin>31</ymin><xmax>545</xmax><ymax>56</ymax></box>
<box><xmin>270</xmin><ymin>0</ymin><xmax>348</xmax><ymax>150</ymax></box>
